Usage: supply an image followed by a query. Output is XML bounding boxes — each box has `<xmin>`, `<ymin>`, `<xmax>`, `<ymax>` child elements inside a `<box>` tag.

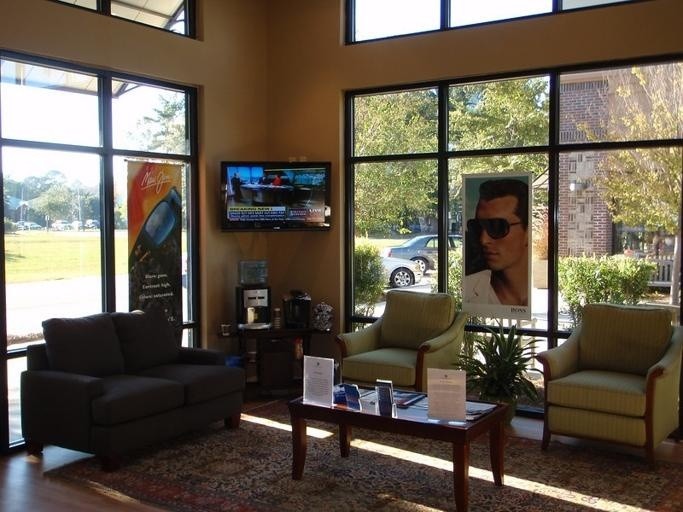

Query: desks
<box><xmin>217</xmin><ymin>328</ymin><xmax>310</xmax><ymax>397</ymax></box>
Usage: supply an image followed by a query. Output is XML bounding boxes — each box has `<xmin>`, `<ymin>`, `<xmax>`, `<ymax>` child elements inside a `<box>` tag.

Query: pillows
<box><xmin>42</xmin><ymin>312</ymin><xmax>124</xmax><ymax>378</ymax></box>
<box><xmin>111</xmin><ymin>302</ymin><xmax>180</xmax><ymax>373</ymax></box>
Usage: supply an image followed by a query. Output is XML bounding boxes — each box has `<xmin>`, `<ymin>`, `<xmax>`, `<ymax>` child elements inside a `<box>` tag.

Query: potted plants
<box><xmin>450</xmin><ymin>321</ymin><xmax>545</xmax><ymax>425</ymax></box>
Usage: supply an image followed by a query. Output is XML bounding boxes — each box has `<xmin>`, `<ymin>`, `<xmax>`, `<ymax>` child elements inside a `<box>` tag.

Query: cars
<box><xmin>24</xmin><ymin>223</ymin><xmax>42</xmax><ymax>230</ymax></box>
<box><xmin>52</xmin><ymin>220</ymin><xmax>74</xmax><ymax>231</ymax></box>
<box><xmin>368</xmin><ymin>257</ymin><xmax>423</xmax><ymax>288</ymax></box>
<box><xmin>380</xmin><ymin>235</ymin><xmax>462</xmax><ymax>274</ymax></box>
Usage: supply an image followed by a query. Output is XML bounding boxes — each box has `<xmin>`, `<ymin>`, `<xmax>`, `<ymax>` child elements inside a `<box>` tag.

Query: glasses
<box><xmin>467</xmin><ymin>218</ymin><xmax>521</xmax><ymax>240</ymax></box>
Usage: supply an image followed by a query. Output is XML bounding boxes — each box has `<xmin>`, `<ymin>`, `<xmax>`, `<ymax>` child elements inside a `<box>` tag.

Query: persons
<box><xmin>230</xmin><ymin>172</ymin><xmax>242</xmax><ymax>203</ymax></box>
<box><xmin>258</xmin><ymin>172</ymin><xmax>268</xmax><ymax>185</ymax></box>
<box><xmin>270</xmin><ymin>174</ymin><xmax>281</xmax><ymax>185</ymax></box>
<box><xmin>467</xmin><ymin>177</ymin><xmax>528</xmax><ymax>305</ymax></box>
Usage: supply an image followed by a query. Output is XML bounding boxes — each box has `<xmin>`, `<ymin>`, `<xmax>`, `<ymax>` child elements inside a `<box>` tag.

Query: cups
<box><xmin>272</xmin><ymin>307</ymin><xmax>281</xmax><ymax>332</ymax></box>
<box><xmin>220</xmin><ymin>323</ymin><xmax>233</xmax><ymax>336</ymax></box>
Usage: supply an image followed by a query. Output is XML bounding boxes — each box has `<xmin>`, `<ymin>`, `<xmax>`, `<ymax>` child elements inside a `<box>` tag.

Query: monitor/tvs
<box><xmin>220</xmin><ymin>161</ymin><xmax>333</xmax><ymax>232</ymax></box>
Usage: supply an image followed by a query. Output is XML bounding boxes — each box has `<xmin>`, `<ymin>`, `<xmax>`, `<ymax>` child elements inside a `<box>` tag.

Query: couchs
<box><xmin>536</xmin><ymin>304</ymin><xmax>682</xmax><ymax>470</ymax></box>
<box><xmin>334</xmin><ymin>290</ymin><xmax>467</xmax><ymax>392</ymax></box>
<box><xmin>22</xmin><ymin>308</ymin><xmax>246</xmax><ymax>472</ymax></box>
